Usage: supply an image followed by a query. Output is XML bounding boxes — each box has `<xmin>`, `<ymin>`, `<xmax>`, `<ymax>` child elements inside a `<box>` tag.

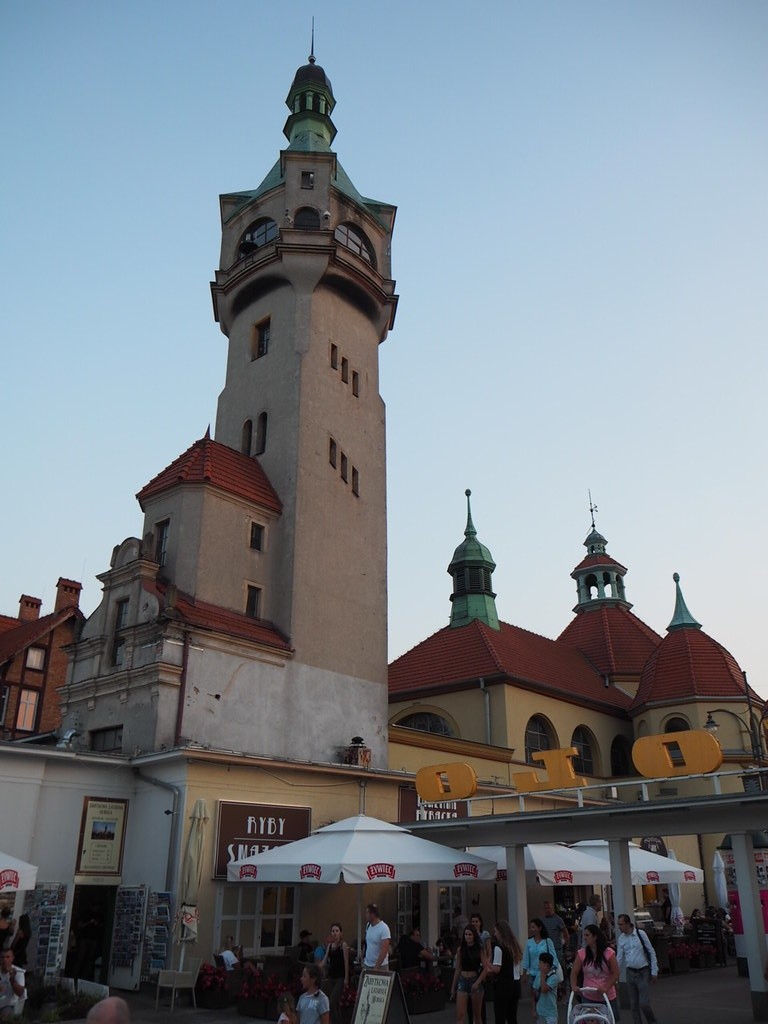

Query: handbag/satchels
<box><xmin>338</xmin><ymin>987</ymin><xmax>354</xmax><ymax>1008</ymax></box>
<box><xmin>637</xmin><ymin>927</ymin><xmax>651</xmax><ymax>961</ymax></box>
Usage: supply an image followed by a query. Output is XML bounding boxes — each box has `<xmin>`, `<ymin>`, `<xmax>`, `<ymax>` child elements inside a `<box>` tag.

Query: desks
<box><xmin>413</xmin><ymin>955</ymin><xmax>454</xmax><ymax>983</ymax></box>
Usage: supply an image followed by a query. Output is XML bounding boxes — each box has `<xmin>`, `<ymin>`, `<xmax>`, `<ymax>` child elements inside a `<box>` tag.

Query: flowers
<box><xmin>666</xmin><ymin>941</ymin><xmax>716</xmax><ymax>958</ymax></box>
<box><xmin>399</xmin><ymin>971</ymin><xmax>445</xmax><ymax>999</ymax></box>
<box><xmin>233</xmin><ymin>963</ymin><xmax>300</xmax><ymax>1002</ymax></box>
<box><xmin>338</xmin><ymin>989</ymin><xmax>359</xmax><ymax>1007</ymax></box>
<box><xmin>194</xmin><ymin>964</ymin><xmax>231</xmax><ymax>993</ymax></box>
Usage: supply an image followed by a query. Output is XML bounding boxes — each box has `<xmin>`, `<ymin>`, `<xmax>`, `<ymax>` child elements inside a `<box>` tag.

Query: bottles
<box><xmin>440</xmin><ymin>943</ymin><xmax>443</xmax><ymax>952</ymax></box>
<box><xmin>433</xmin><ymin>947</ymin><xmax>436</xmax><ymax>957</ymax></box>
<box><xmin>423</xmin><ymin>943</ymin><xmax>426</xmax><ymax>949</ymax></box>
<box><xmin>437</xmin><ymin>947</ymin><xmax>439</xmax><ymax>957</ymax></box>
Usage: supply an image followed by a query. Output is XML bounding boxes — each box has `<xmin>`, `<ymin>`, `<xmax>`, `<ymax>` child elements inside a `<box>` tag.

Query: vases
<box><xmin>237</xmin><ymin>995</ymin><xmax>280</xmax><ymax>1021</ymax></box>
<box><xmin>668</xmin><ymin>955</ymin><xmax>689</xmax><ymax>974</ymax></box>
<box><xmin>691</xmin><ymin>953</ymin><xmax>709</xmax><ymax>969</ymax></box>
<box><xmin>406</xmin><ymin>990</ymin><xmax>444</xmax><ymax>1015</ymax></box>
<box><xmin>341</xmin><ymin>1005</ymin><xmax>354</xmax><ymax>1024</ymax></box>
<box><xmin>194</xmin><ymin>989</ymin><xmax>230</xmax><ymax>1010</ymax></box>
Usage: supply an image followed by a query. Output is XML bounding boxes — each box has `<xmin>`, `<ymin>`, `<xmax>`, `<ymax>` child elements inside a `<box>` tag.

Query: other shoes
<box><xmin>561</xmin><ymin>1001</ymin><xmax>568</xmax><ymax>1006</ymax></box>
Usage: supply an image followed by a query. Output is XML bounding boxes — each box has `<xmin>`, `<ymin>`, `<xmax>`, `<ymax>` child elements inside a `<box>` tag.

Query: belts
<box><xmin>628</xmin><ymin>966</ymin><xmax>647</xmax><ymax>972</ymax></box>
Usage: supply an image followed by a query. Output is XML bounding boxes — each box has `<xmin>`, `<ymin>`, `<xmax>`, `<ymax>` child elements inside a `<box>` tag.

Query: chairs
<box><xmin>155</xmin><ymin>969</ymin><xmax>197</xmax><ymax>1013</ymax></box>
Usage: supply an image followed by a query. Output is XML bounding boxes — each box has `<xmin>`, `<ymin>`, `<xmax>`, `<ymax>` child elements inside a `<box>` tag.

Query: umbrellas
<box><xmin>667</xmin><ymin>848</ymin><xmax>687</xmax><ymax>934</ymax></box>
<box><xmin>170</xmin><ymin>799</ymin><xmax>210</xmax><ymax>1000</ymax></box>
<box><xmin>0</xmin><ymin>848</ymin><xmax>39</xmax><ymax>893</ymax></box>
<box><xmin>227</xmin><ymin>814</ymin><xmax>704</xmax><ymax>959</ymax></box>
<box><xmin>711</xmin><ymin>849</ymin><xmax>729</xmax><ymax>916</ymax></box>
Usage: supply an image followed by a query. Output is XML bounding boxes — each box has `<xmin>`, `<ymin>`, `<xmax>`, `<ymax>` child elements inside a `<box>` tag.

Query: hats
<box><xmin>539</xmin><ymin>953</ymin><xmax>558</xmax><ymax>970</ymax></box>
<box><xmin>299</xmin><ymin>930</ymin><xmax>312</xmax><ymax>938</ymax></box>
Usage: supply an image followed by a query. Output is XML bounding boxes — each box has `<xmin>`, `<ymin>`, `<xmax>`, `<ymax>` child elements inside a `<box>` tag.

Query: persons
<box><xmin>0</xmin><ymin>907</ymin><xmax>32</xmax><ymax>1024</ymax></box>
<box><xmin>315</xmin><ymin>922</ymin><xmax>350</xmax><ymax>1024</ymax></box>
<box><xmin>219</xmin><ymin>935</ymin><xmax>259</xmax><ymax>975</ymax></box>
<box><xmin>294</xmin><ymin>893</ymin><xmax>736</xmax><ymax>990</ymax></box>
<box><xmin>86</xmin><ymin>996</ymin><xmax>130</xmax><ymax>1024</ymax></box>
<box><xmin>449</xmin><ymin>914</ymin><xmax>658</xmax><ymax>1024</ymax></box>
<box><xmin>277</xmin><ymin>962</ymin><xmax>330</xmax><ymax>1024</ymax></box>
